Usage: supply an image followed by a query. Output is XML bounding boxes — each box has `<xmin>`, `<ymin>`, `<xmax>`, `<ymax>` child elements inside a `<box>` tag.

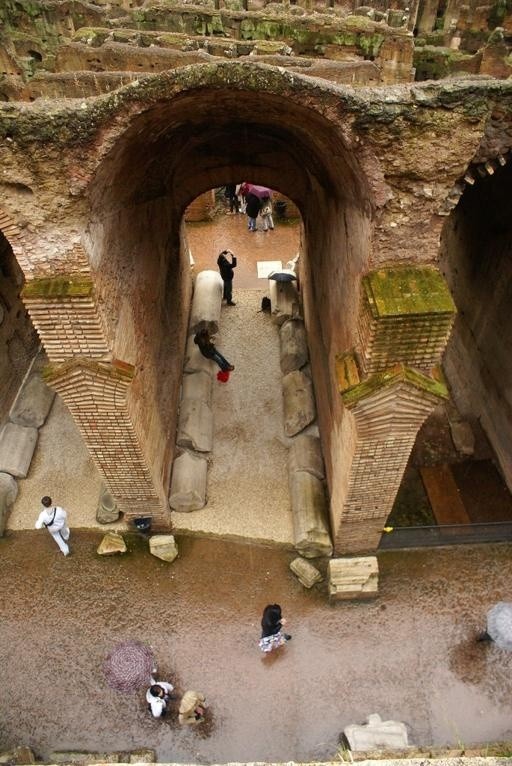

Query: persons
<box><xmin>238</xmin><ymin>183</ymin><xmax>249</xmax><ymax>214</ymax></box>
<box><xmin>257</xmin><ymin>602</ymin><xmax>291</xmax><ymax>654</ymax></box>
<box><xmin>475</xmin><ymin>631</ymin><xmax>492</xmax><ymax>643</ymax></box>
<box><xmin>259</xmin><ymin>196</ymin><xmax>275</xmax><ymax>231</ymax></box>
<box><xmin>245</xmin><ymin>192</ymin><xmax>264</xmax><ymax>232</ymax></box>
<box><xmin>224</xmin><ymin>184</ymin><xmax>240</xmax><ymax>215</ymax></box>
<box><xmin>144</xmin><ymin>681</ymin><xmax>177</xmax><ymax>721</ymax></box>
<box><xmin>217</xmin><ymin>249</ymin><xmax>237</xmax><ymax>306</ymax></box>
<box><xmin>34</xmin><ymin>495</ymin><xmax>72</xmax><ymax>557</ymax></box>
<box><xmin>194</xmin><ymin>328</ymin><xmax>235</xmax><ymax>371</ymax></box>
<box><xmin>176</xmin><ymin>689</ymin><xmax>207</xmax><ymax>726</ymax></box>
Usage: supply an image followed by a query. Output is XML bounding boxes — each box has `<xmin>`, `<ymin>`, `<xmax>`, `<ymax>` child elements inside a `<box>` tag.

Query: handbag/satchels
<box><xmin>260</xmin><ymin>206</ymin><xmax>272</xmax><ymax>217</ymax></box>
<box><xmin>257</xmin><ymin>630</ymin><xmax>287</xmax><ymax>653</ymax></box>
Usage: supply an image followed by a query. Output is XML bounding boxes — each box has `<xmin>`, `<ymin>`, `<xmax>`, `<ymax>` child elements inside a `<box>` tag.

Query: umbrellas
<box><xmin>248</xmin><ymin>184</ymin><xmax>273</xmax><ymax>199</ymax></box>
<box><xmin>100</xmin><ymin>638</ymin><xmax>157</xmax><ymax>691</ymax></box>
<box><xmin>485</xmin><ymin>601</ymin><xmax>512</xmax><ymax>651</ymax></box>
<box><xmin>267</xmin><ymin>270</ymin><xmax>298</xmax><ymax>291</ymax></box>
